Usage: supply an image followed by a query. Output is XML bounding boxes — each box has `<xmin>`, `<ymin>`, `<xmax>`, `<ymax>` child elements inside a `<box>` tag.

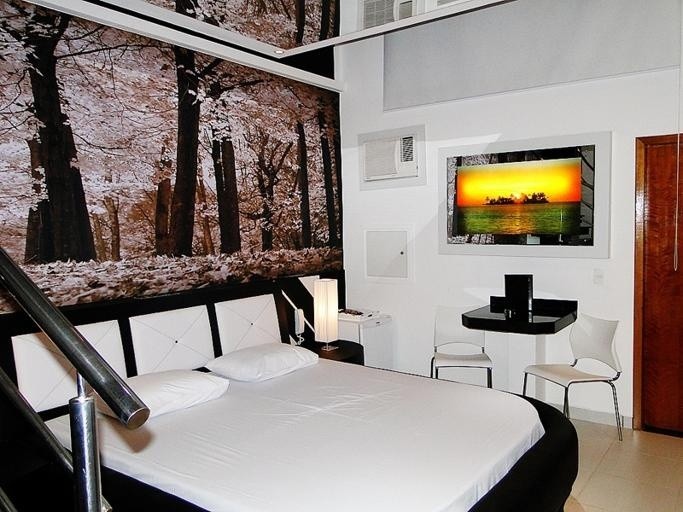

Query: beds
<box><xmin>1</xmin><ymin>297</ymin><xmax>579</xmax><ymax>512</ymax></box>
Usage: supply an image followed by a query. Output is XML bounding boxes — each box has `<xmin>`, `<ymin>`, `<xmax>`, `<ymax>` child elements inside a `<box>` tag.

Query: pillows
<box><xmin>95</xmin><ymin>339</ymin><xmax>319</xmax><ymax>424</ymax></box>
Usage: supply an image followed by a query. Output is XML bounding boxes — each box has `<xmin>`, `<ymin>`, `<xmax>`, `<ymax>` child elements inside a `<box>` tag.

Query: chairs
<box><xmin>521</xmin><ymin>313</ymin><xmax>624</xmax><ymax>442</ymax></box>
<box><xmin>431</xmin><ymin>301</ymin><xmax>493</xmax><ymax>386</ymax></box>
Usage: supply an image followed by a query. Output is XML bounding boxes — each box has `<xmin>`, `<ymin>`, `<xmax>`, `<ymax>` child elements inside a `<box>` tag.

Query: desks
<box><xmin>463</xmin><ymin>297</ymin><xmax>579</xmax><ymax>397</ymax></box>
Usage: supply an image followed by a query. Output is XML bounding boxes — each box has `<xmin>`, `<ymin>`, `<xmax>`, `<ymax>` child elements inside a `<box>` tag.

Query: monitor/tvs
<box><xmin>447</xmin><ymin>144</ymin><xmax>595</xmax><ymax>246</ymax></box>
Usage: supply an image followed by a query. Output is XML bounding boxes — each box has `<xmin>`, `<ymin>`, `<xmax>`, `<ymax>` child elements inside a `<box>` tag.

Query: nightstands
<box><xmin>316</xmin><ymin>341</ymin><xmax>365</xmax><ymax>364</ymax></box>
